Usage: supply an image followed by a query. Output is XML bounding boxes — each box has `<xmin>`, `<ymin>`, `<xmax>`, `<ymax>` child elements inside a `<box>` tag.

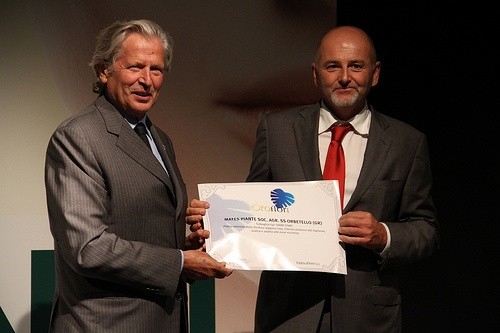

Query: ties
<box><xmin>134</xmin><ymin>122</ymin><xmax>152</xmax><ymax>152</ymax></box>
<box><xmin>322</xmin><ymin>123</ymin><xmax>354</xmax><ymax>212</ymax></box>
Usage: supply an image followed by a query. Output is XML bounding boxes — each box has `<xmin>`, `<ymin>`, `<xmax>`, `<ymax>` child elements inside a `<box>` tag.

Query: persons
<box><xmin>45</xmin><ymin>19</ymin><xmax>231</xmax><ymax>333</ymax></box>
<box><xmin>186</xmin><ymin>26</ymin><xmax>440</xmax><ymax>333</ymax></box>
